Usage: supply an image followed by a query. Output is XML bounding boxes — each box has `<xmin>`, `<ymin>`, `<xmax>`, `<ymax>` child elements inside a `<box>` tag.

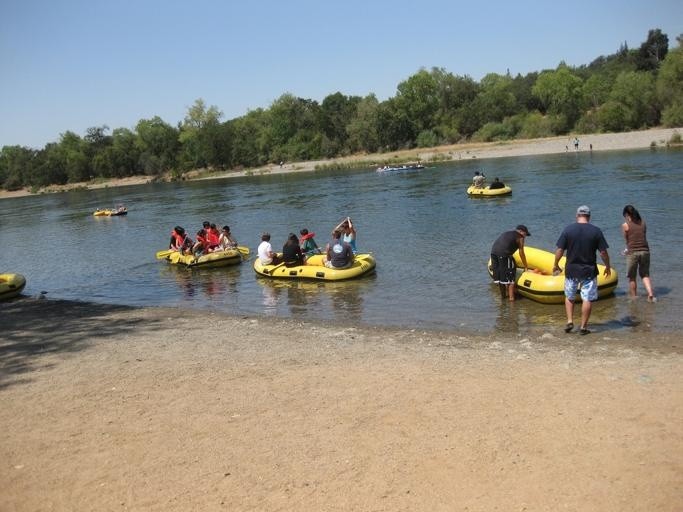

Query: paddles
<box><xmin>156</xmin><ymin>249</ymin><xmax>179</xmax><ymax>259</ymax></box>
<box><xmin>237</xmin><ymin>246</ymin><xmax>249</xmax><ymax>254</ymax></box>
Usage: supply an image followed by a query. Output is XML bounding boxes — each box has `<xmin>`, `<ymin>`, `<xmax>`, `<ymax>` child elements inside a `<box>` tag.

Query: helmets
<box><xmin>174</xmin><ymin>221</ymin><xmax>216</xmax><ymax>235</ymax></box>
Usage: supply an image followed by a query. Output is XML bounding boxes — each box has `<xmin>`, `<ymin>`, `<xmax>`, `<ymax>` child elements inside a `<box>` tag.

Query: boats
<box><xmin>375</xmin><ymin>164</ymin><xmax>424</xmax><ymax>172</ymax></box>
<box><xmin>465</xmin><ymin>185</ymin><xmax>511</xmax><ymax>196</ymax></box>
<box><xmin>253</xmin><ymin>252</ymin><xmax>376</xmax><ymax>282</ymax></box>
<box><xmin>165</xmin><ymin>247</ymin><xmax>241</xmax><ymax>268</ymax></box>
<box><xmin>92</xmin><ymin>210</ymin><xmax>127</xmax><ymax>216</ymax></box>
<box><xmin>254</xmin><ymin>274</ymin><xmax>369</xmax><ymax>293</ymax></box>
<box><xmin>486</xmin><ymin>246</ymin><xmax>617</xmax><ymax>304</ymax></box>
<box><xmin>486</xmin><ymin>280</ymin><xmax>617</xmax><ymax>324</ymax></box>
<box><xmin>0</xmin><ymin>273</ymin><xmax>26</xmax><ymax>299</ymax></box>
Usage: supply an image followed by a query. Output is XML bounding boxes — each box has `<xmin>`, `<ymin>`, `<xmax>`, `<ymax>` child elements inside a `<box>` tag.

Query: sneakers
<box><xmin>564</xmin><ymin>322</ymin><xmax>590</xmax><ymax>335</ymax></box>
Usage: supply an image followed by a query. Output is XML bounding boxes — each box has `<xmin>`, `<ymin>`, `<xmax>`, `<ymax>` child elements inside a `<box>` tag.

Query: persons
<box><xmin>299</xmin><ymin>228</ymin><xmax>322</xmax><ymax>255</ymax></box>
<box><xmin>336</xmin><ymin>216</ymin><xmax>358</xmax><ymax>260</ymax></box>
<box><xmin>473</xmin><ymin>171</ymin><xmax>487</xmax><ymax>189</ymax></box>
<box><xmin>572</xmin><ymin>137</ymin><xmax>580</xmax><ymax>150</ymax></box>
<box><xmin>322</xmin><ymin>230</ymin><xmax>354</xmax><ymax>271</ymax></box>
<box><xmin>282</xmin><ymin>233</ymin><xmax>313</xmax><ymax>268</ymax></box>
<box><xmin>490</xmin><ymin>225</ymin><xmax>532</xmax><ymax>302</ymax></box>
<box><xmin>169</xmin><ymin>220</ymin><xmax>238</xmax><ymax>257</ymax></box>
<box><xmin>258</xmin><ymin>233</ymin><xmax>283</xmax><ymax>265</ymax></box>
<box><xmin>279</xmin><ymin>160</ymin><xmax>283</xmax><ymax>167</ymax></box>
<box><xmin>552</xmin><ymin>204</ymin><xmax>612</xmax><ymax>336</ymax></box>
<box><xmin>621</xmin><ymin>205</ymin><xmax>654</xmax><ymax>301</ymax></box>
<box><xmin>119</xmin><ymin>206</ymin><xmax>127</xmax><ymax>213</ymax></box>
<box><xmin>485</xmin><ymin>177</ymin><xmax>505</xmax><ymax>189</ymax></box>
<box><xmin>383</xmin><ymin>162</ymin><xmax>389</xmax><ymax>171</ymax></box>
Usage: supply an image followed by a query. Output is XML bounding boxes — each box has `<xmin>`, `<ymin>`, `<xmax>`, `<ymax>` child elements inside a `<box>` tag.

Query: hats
<box><xmin>516</xmin><ymin>225</ymin><xmax>531</xmax><ymax>236</ymax></box>
<box><xmin>577</xmin><ymin>205</ymin><xmax>590</xmax><ymax>214</ymax></box>
